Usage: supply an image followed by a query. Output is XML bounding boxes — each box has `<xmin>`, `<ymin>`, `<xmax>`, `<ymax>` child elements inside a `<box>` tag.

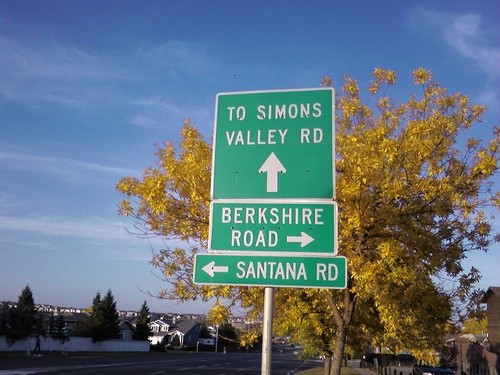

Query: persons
<box><xmin>33</xmin><ymin>335</ymin><xmax>41</xmax><ymax>353</ymax></box>
